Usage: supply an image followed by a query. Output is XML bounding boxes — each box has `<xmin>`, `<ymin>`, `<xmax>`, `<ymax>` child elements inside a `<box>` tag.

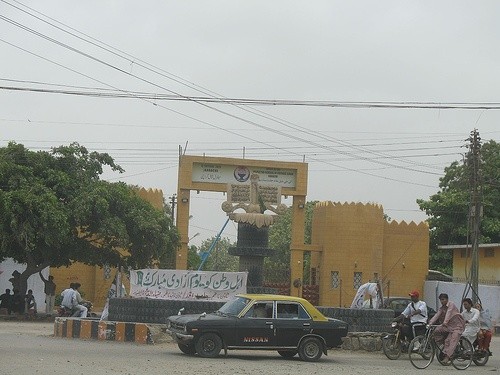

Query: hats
<box><xmin>408</xmin><ymin>291</ymin><xmax>418</xmax><ymax>297</ymax></box>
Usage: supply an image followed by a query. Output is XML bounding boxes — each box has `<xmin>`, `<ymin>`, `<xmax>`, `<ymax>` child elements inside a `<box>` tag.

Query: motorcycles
<box><xmin>56</xmin><ymin>301</ymin><xmax>98</xmax><ymax>317</ymax></box>
<box><xmin>437</xmin><ymin>320</ymin><xmax>490</xmax><ymax>367</ymax></box>
<box><xmin>383</xmin><ymin>315</ymin><xmax>434</xmax><ymax>361</ymax></box>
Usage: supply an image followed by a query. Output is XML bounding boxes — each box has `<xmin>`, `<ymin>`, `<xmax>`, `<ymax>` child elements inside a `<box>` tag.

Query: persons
<box><xmin>473</xmin><ymin>304</ymin><xmax>494</xmax><ymax>354</ymax></box>
<box><xmin>0</xmin><ymin>270</ymin><xmax>126</xmax><ymax>318</ymax></box>
<box><xmin>426</xmin><ymin>293</ymin><xmax>465</xmax><ymax>364</ymax></box>
<box><xmin>458</xmin><ymin>298</ymin><xmax>482</xmax><ymax>354</ymax></box>
<box><xmin>394</xmin><ymin>290</ymin><xmax>428</xmax><ymax>351</ymax></box>
<box><xmin>252</xmin><ymin>304</ymin><xmax>298</xmax><ymax>318</ymax></box>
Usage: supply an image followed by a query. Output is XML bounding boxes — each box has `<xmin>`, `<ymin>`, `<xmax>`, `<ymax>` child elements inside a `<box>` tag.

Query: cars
<box><xmin>362</xmin><ymin>295</ymin><xmax>438</xmax><ymax>319</ymax></box>
<box><xmin>167</xmin><ymin>294</ymin><xmax>350</xmax><ymax>363</ymax></box>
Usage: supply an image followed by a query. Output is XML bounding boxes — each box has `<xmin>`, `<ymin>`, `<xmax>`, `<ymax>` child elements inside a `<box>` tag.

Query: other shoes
<box><xmin>440</xmin><ymin>357</ymin><xmax>448</xmax><ymax>365</ymax></box>
<box><xmin>413</xmin><ymin>341</ymin><xmax>422</xmax><ymax>350</ymax></box>
<box><xmin>477</xmin><ymin>348</ymin><xmax>490</xmax><ymax>354</ymax></box>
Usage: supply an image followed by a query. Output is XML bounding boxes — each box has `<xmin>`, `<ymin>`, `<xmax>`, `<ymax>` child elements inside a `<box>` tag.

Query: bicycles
<box><xmin>408</xmin><ymin>321</ymin><xmax>474</xmax><ymax>370</ymax></box>
